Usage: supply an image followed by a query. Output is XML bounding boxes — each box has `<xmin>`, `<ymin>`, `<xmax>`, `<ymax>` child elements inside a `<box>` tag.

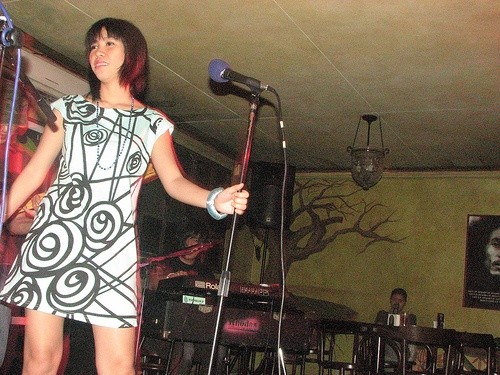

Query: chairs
<box><xmin>299</xmin><ymin>317</ymin><xmax>500</xmax><ymax>375</ymax></box>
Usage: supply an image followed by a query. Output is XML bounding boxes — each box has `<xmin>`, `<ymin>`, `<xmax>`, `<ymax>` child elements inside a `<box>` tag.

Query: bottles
<box><xmin>389</xmin><ymin>314</ymin><xmax>394</xmax><ymax>325</ymax></box>
<box><xmin>393</xmin><ymin>303</ymin><xmax>399</xmax><ymax>314</ymax></box>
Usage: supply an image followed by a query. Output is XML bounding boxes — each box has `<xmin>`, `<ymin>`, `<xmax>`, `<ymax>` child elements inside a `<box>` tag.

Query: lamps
<box><xmin>347</xmin><ymin>112</ymin><xmax>390</xmax><ymax>191</ymax></box>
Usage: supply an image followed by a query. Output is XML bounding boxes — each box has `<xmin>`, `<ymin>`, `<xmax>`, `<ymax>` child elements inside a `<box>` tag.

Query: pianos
<box><xmin>143</xmin><ymin>275</ymin><xmax>308</xmax><ymax>375</ymax></box>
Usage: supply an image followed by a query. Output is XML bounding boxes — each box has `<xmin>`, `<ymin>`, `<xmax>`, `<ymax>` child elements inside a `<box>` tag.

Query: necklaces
<box><xmin>96</xmin><ymin>94</ymin><xmax>134</xmax><ymax>170</ymax></box>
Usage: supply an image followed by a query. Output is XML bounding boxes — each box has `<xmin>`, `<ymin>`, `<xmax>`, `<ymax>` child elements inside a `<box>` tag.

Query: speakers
<box><xmin>250</xmin><ymin>160</ymin><xmax>295</xmax><ymax>229</ymax></box>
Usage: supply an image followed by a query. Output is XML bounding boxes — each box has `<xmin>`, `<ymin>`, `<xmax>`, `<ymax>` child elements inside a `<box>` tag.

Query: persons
<box><xmin>1</xmin><ymin>18</ymin><xmax>250</xmax><ymax>375</ymax></box>
<box><xmin>355</xmin><ymin>288</ymin><xmax>417</xmax><ymax>374</ymax></box>
<box><xmin>147</xmin><ymin>221</ymin><xmax>235</xmax><ymax>375</ymax></box>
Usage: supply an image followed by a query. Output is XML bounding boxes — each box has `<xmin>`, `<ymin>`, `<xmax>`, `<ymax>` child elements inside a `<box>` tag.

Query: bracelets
<box><xmin>206</xmin><ymin>187</ymin><xmax>228</xmax><ymax>221</ymax></box>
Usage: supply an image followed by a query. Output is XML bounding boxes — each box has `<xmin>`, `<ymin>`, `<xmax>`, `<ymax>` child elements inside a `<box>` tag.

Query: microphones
<box><xmin>209</xmin><ymin>59</ymin><xmax>268</xmax><ymax>91</ymax></box>
